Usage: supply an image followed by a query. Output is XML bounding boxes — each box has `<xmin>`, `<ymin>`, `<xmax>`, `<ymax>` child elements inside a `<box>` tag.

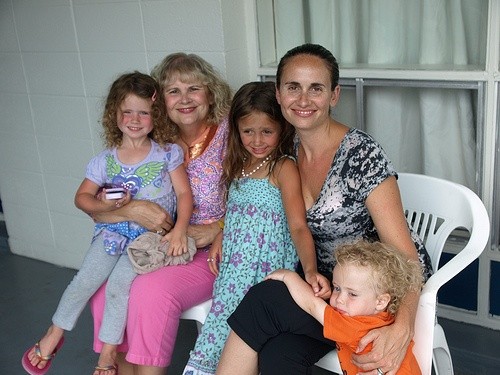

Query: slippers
<box><xmin>93</xmin><ymin>364</ymin><xmax>118</xmax><ymax>375</ymax></box>
<box><xmin>21</xmin><ymin>336</ymin><xmax>64</xmax><ymax>375</ymax></box>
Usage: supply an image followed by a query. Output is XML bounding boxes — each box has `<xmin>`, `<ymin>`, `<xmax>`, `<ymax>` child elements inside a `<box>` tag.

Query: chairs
<box><xmin>179</xmin><ymin>174</ymin><xmax>492</xmax><ymax>375</ymax></box>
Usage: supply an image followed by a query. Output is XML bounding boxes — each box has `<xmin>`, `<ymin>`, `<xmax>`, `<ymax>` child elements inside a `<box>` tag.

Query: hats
<box><xmin>127</xmin><ymin>230</ymin><xmax>196</xmax><ymax>274</ymax></box>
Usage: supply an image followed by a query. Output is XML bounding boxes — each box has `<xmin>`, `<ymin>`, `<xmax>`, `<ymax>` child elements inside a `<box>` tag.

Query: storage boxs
<box><xmin>105</xmin><ymin>188</ymin><xmax>126</xmax><ymax>201</ymax></box>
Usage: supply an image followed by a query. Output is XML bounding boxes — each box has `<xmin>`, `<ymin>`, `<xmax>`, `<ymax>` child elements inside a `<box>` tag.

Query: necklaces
<box><xmin>241</xmin><ymin>150</ymin><xmax>274</xmax><ymax>177</ymax></box>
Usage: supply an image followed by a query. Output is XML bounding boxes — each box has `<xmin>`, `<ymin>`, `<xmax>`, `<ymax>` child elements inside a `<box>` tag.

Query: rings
<box><xmin>157</xmin><ymin>228</ymin><xmax>164</xmax><ymax>234</ymax></box>
<box><xmin>115</xmin><ymin>200</ymin><xmax>120</xmax><ymax>206</ymax></box>
<box><xmin>377</xmin><ymin>368</ymin><xmax>385</xmax><ymax>375</ymax></box>
<box><xmin>207</xmin><ymin>258</ymin><xmax>217</xmax><ymax>262</ymax></box>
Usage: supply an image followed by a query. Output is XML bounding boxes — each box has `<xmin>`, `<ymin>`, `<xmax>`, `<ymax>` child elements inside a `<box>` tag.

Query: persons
<box><xmin>87</xmin><ymin>52</ymin><xmax>233</xmax><ymax>375</ymax></box>
<box><xmin>22</xmin><ymin>71</ymin><xmax>193</xmax><ymax>375</ymax></box>
<box><xmin>214</xmin><ymin>43</ymin><xmax>433</xmax><ymax>375</ymax></box>
<box><xmin>182</xmin><ymin>81</ymin><xmax>332</xmax><ymax>375</ymax></box>
<box><xmin>263</xmin><ymin>240</ymin><xmax>424</xmax><ymax>375</ymax></box>
